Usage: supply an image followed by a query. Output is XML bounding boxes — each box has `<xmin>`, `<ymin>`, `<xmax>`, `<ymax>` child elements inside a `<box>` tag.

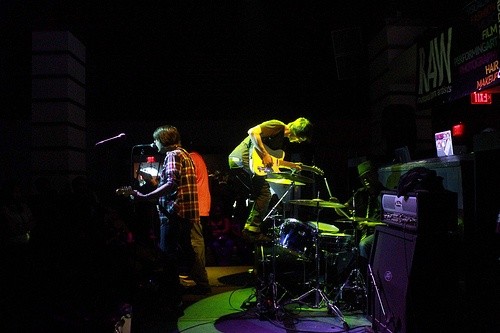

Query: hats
<box><xmin>356</xmin><ymin>161</ymin><xmax>373</xmax><ymax>177</ymax></box>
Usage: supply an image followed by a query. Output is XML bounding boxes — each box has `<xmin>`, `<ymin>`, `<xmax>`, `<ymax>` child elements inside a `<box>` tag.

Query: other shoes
<box><xmin>242</xmin><ymin>230</ymin><xmax>270</xmax><ymax>245</ymax></box>
<box><xmin>188</xmin><ymin>283</ymin><xmax>213</xmax><ymax>295</ymax></box>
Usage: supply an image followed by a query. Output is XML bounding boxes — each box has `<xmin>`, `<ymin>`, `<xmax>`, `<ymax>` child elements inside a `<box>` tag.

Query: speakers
<box><xmin>368</xmin><ymin>226</ymin><xmax>458</xmax><ymax>332</ymax></box>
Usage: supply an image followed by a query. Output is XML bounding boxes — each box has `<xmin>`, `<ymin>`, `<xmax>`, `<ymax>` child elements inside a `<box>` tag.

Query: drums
<box><xmin>265</xmin><ymin>224</ymin><xmax>279</xmax><ymax>241</ymax></box>
<box><xmin>308</xmin><ymin>220</ymin><xmax>340</xmax><ymax>250</ymax></box>
<box><xmin>317</xmin><ymin>231</ymin><xmax>354</xmax><ymax>255</ymax></box>
<box><xmin>275</xmin><ymin>218</ymin><xmax>312</xmax><ymax>263</ymax></box>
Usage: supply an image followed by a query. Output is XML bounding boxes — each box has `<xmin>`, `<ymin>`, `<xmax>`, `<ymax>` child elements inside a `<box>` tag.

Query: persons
<box><xmin>207</xmin><ymin>205</ymin><xmax>230</xmax><ymax>239</ymax></box>
<box><xmin>130</xmin><ymin>126</ymin><xmax>211</xmax><ymax>306</ymax></box>
<box><xmin>227</xmin><ymin>116</ymin><xmax>313</xmax><ymax>240</ymax></box>
<box><xmin>0</xmin><ymin>173</ymin><xmax>99</xmax><ymax>246</ymax></box>
<box><xmin>329</xmin><ymin>161</ymin><xmax>391</xmax><ymax>261</ymax></box>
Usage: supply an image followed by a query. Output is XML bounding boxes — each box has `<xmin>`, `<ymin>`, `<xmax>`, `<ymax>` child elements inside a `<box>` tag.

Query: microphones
<box><xmin>136</xmin><ymin>143</ymin><xmax>156</xmax><ymax>148</ymax></box>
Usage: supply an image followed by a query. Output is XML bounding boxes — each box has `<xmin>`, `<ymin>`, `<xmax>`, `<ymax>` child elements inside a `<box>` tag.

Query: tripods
<box><xmin>241</xmin><ymin>202</ymin><xmax>376</xmax><ymax>328</ymax></box>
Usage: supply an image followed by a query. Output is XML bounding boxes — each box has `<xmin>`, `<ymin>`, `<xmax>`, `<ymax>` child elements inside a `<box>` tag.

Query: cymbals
<box><xmin>334</xmin><ymin>218</ymin><xmax>375</xmax><ymax>223</ymax></box>
<box><xmin>263</xmin><ymin>170</ymin><xmax>316</xmax><ymax>185</ymax></box>
<box><xmin>289</xmin><ymin>199</ymin><xmax>345</xmax><ymax>208</ymax></box>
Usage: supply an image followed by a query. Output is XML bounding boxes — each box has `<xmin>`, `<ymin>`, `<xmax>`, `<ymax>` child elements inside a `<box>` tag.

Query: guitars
<box><xmin>115</xmin><ymin>184</ymin><xmax>159</xmax><ymax>205</ymax></box>
<box><xmin>248</xmin><ymin>143</ymin><xmax>325</xmax><ymax>177</ymax></box>
